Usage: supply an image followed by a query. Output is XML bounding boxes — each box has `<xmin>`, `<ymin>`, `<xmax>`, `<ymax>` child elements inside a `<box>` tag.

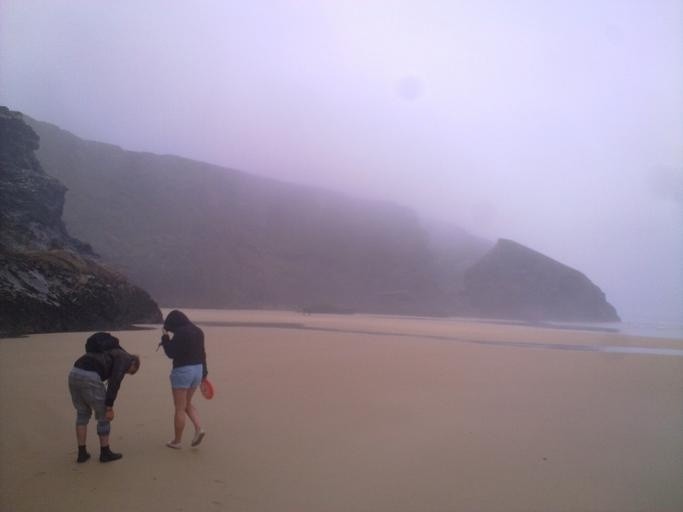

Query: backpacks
<box><xmin>86</xmin><ymin>332</ymin><xmax>126</xmax><ymax>354</ymax></box>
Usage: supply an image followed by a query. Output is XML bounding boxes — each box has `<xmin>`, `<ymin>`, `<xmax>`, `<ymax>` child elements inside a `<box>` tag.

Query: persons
<box><xmin>160</xmin><ymin>309</ymin><xmax>207</xmax><ymax>449</ymax></box>
<box><xmin>66</xmin><ymin>347</ymin><xmax>140</xmax><ymax>464</ymax></box>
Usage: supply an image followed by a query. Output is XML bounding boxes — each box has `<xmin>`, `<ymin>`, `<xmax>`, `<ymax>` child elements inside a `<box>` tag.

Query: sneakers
<box><xmin>167</xmin><ymin>440</ymin><xmax>182</xmax><ymax>448</ymax></box>
<box><xmin>78</xmin><ymin>452</ymin><xmax>91</xmax><ymax>462</ymax></box>
<box><xmin>191</xmin><ymin>429</ymin><xmax>205</xmax><ymax>446</ymax></box>
<box><xmin>100</xmin><ymin>451</ymin><xmax>121</xmax><ymax>461</ymax></box>
